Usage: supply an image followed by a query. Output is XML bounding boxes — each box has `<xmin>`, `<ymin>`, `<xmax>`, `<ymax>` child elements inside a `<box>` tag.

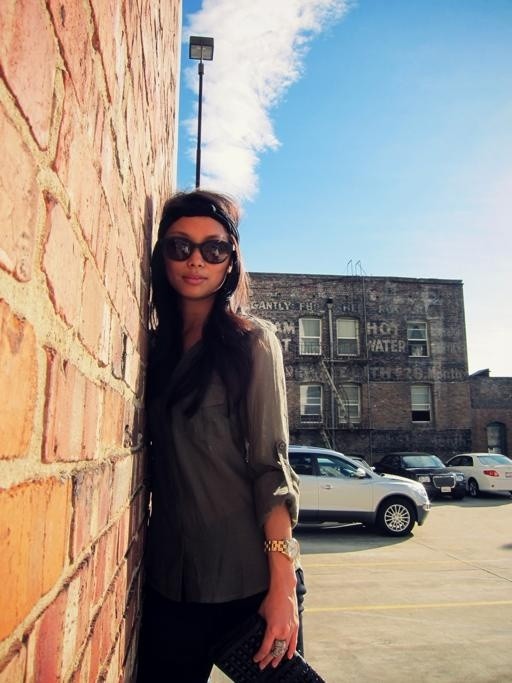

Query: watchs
<box><xmin>264</xmin><ymin>536</ymin><xmax>300</xmax><ymax>561</ymax></box>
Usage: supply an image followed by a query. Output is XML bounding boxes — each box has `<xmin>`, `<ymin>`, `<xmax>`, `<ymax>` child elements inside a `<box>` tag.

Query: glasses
<box><xmin>158</xmin><ymin>237</ymin><xmax>235</xmax><ymax>263</ymax></box>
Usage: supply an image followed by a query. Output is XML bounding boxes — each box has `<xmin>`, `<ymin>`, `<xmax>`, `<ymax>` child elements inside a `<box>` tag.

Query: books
<box><xmin>212</xmin><ymin>612</ymin><xmax>325</xmax><ymax>683</ymax></box>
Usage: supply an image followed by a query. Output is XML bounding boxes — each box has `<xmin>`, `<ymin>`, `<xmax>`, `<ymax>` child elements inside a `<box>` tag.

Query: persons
<box><xmin>141</xmin><ymin>186</ymin><xmax>307</xmax><ymax>683</ymax></box>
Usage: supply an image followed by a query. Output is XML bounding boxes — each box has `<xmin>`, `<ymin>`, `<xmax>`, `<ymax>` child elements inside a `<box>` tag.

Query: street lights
<box><xmin>189</xmin><ymin>36</ymin><xmax>213</xmax><ymax>190</ymax></box>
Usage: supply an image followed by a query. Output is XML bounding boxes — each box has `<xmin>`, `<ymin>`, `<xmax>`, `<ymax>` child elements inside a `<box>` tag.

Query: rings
<box><xmin>272</xmin><ymin>640</ymin><xmax>287</xmax><ymax>657</ymax></box>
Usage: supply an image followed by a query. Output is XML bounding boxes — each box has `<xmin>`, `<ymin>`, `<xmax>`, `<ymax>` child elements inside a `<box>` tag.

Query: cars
<box><xmin>444</xmin><ymin>452</ymin><xmax>512</xmax><ymax>496</ymax></box>
<box><xmin>373</xmin><ymin>452</ymin><xmax>466</xmax><ymax>500</ymax></box>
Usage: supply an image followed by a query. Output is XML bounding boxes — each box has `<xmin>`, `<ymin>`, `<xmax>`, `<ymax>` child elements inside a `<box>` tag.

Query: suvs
<box><xmin>288</xmin><ymin>444</ymin><xmax>430</xmax><ymax>536</ymax></box>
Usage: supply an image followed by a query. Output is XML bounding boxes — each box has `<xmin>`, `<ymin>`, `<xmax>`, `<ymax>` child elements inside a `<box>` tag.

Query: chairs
<box><xmin>302</xmin><ymin>460</ymin><xmax>313</xmax><ymax>475</ymax></box>
